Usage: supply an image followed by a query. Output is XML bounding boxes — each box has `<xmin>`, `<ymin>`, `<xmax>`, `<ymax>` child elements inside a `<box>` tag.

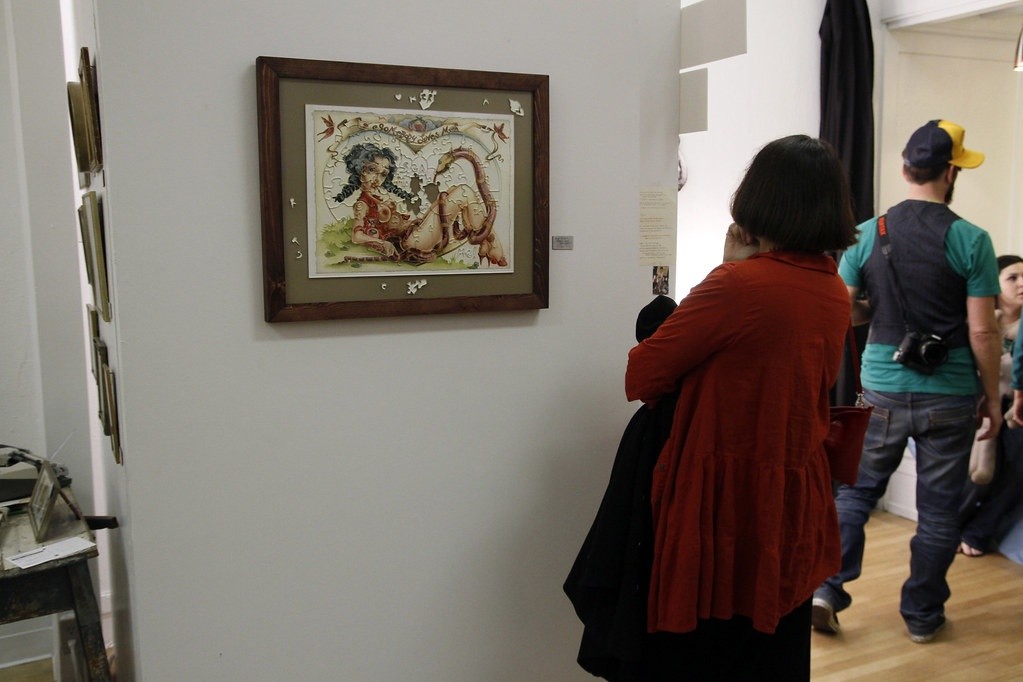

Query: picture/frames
<box><xmin>66</xmin><ymin>48</ymin><xmax>123</xmax><ymax>464</ymax></box>
<box><xmin>25</xmin><ymin>461</ymin><xmax>83</xmax><ymax>544</ymax></box>
<box><xmin>255</xmin><ymin>55</ymin><xmax>551</xmax><ymax>324</ymax></box>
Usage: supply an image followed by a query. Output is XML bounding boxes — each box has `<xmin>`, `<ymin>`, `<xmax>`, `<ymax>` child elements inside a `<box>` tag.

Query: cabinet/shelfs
<box><xmin>0</xmin><ymin>487</ymin><xmax>114</xmax><ymax>682</ymax></box>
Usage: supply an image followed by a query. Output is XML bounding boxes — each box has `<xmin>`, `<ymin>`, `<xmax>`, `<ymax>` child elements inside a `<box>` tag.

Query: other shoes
<box><xmin>909</xmin><ymin>612</ymin><xmax>945</xmax><ymax>641</ymax></box>
<box><xmin>811</xmin><ymin>588</ymin><xmax>840</xmax><ymax>635</ymax></box>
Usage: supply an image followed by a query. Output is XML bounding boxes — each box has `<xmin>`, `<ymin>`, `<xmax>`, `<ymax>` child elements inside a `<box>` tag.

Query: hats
<box><xmin>902</xmin><ymin>120</ymin><xmax>985</xmax><ymax>168</ymax></box>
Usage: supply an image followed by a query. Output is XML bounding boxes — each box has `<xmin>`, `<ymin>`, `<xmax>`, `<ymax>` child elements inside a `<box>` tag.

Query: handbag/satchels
<box><xmin>826</xmin><ymin>404</ymin><xmax>875</xmax><ymax>486</ymax></box>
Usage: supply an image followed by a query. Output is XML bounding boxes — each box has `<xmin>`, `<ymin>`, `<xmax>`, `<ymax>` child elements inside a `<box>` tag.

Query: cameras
<box><xmin>893</xmin><ymin>329</ymin><xmax>949</xmax><ymax>370</ymax></box>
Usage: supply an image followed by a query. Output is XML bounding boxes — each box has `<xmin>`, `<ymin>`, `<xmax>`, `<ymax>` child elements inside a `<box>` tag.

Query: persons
<box><xmin>954</xmin><ymin>256</ymin><xmax>1023</xmax><ymax>557</ymax></box>
<box><xmin>1003</xmin><ymin>307</ymin><xmax>1023</xmax><ymax>429</ymax></box>
<box><xmin>811</xmin><ymin>118</ymin><xmax>1002</xmax><ymax>644</ymax></box>
<box><xmin>623</xmin><ymin>135</ymin><xmax>862</xmax><ymax>682</ymax></box>
